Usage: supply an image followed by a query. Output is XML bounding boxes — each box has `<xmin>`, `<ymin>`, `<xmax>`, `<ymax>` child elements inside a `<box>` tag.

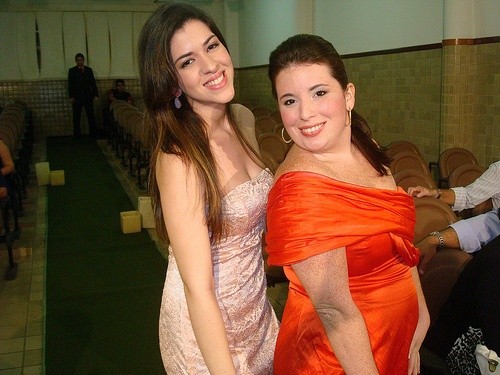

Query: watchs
<box><xmin>428</xmin><ymin>231</ymin><xmax>443</xmax><ymax>251</ymax></box>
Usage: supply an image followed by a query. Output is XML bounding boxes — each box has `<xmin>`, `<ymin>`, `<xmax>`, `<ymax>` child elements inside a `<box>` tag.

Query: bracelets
<box><xmin>434</xmin><ymin>188</ymin><xmax>441</xmax><ymax>199</ymax></box>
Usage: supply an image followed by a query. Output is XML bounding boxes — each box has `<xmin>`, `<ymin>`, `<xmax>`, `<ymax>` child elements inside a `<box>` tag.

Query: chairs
<box><xmin>250</xmin><ymin>106</ymin><xmax>295</xmax><ymax>174</ymax></box>
<box><xmin>386</xmin><ymin>141</ymin><xmax>494</xmax><ymax>324</ymax></box>
<box><xmin>0</xmin><ymin>100</ymin><xmax>35</xmax><ymax>282</ymax></box>
<box><xmin>110</xmin><ymin>97</ymin><xmax>162</xmax><ymax>191</ymax></box>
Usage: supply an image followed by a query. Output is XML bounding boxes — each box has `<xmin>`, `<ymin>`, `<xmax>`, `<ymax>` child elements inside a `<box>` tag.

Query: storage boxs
<box><xmin>36</xmin><ymin>161</ymin><xmax>51</xmax><ymax>187</ymax></box>
<box><xmin>51</xmin><ymin>170</ymin><xmax>65</xmax><ymax>186</ymax></box>
<box><xmin>138</xmin><ymin>197</ymin><xmax>158</xmax><ymax>228</ymax></box>
<box><xmin>120</xmin><ymin>211</ymin><xmax>141</xmax><ymax>234</ymax></box>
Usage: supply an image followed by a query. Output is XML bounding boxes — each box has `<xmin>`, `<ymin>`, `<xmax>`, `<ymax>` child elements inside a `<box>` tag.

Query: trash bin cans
<box><xmin>138</xmin><ymin>196</ymin><xmax>156</xmax><ymax>228</ymax></box>
<box><xmin>50</xmin><ymin>170</ymin><xmax>64</xmax><ymax>186</ymax></box>
<box><xmin>120</xmin><ymin>211</ymin><xmax>141</xmax><ymax>233</ymax></box>
<box><xmin>35</xmin><ymin>162</ymin><xmax>51</xmax><ymax>185</ymax></box>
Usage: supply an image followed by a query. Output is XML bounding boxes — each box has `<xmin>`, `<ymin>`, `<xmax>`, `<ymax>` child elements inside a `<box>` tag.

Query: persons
<box><xmin>414</xmin><ymin>207</ymin><xmax>500</xmax><ymax>274</ymax></box>
<box><xmin>407</xmin><ymin>160</ymin><xmax>500</xmax><ymax>212</ymax></box>
<box><xmin>68</xmin><ymin>53</ymin><xmax>99</xmax><ymax>139</ymax></box>
<box><xmin>137</xmin><ymin>2</ymin><xmax>279</xmax><ymax>375</ymax></box>
<box><xmin>107</xmin><ymin>79</ymin><xmax>132</xmax><ymax>111</ymax></box>
<box><xmin>0</xmin><ymin>140</ymin><xmax>17</xmax><ymax>210</ymax></box>
<box><xmin>266</xmin><ymin>34</ymin><xmax>430</xmax><ymax>375</ymax></box>
